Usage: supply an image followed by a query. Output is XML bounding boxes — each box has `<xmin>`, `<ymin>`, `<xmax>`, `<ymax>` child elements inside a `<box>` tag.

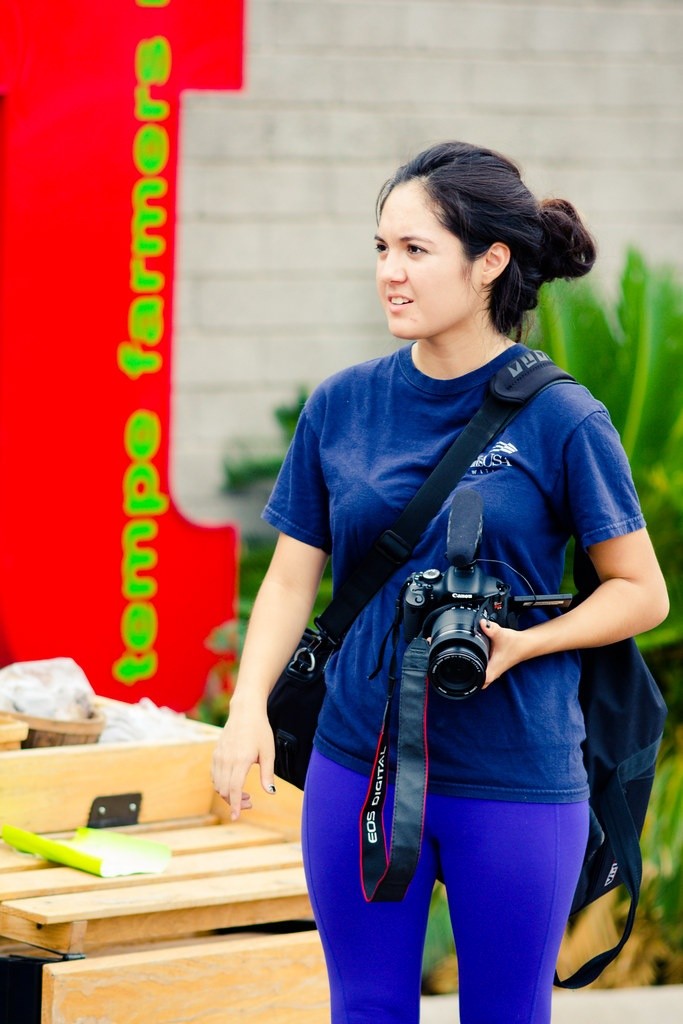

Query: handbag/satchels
<box><xmin>267</xmin><ymin>628</ymin><xmax>335</xmax><ymax>791</ymax></box>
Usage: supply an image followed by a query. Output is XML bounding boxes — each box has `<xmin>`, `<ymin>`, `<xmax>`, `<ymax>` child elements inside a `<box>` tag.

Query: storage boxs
<box><xmin>0</xmin><ymin>693</ymin><xmax>221</xmax><ymax>837</ymax></box>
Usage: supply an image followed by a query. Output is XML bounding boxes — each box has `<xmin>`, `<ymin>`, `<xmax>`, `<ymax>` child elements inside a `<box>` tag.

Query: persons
<box><xmin>208</xmin><ymin>143</ymin><xmax>670</xmax><ymax>1024</ymax></box>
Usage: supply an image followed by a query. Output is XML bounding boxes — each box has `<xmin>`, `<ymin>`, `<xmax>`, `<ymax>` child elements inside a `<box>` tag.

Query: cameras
<box><xmin>403</xmin><ymin>565</ymin><xmax>573</xmax><ymax>701</ymax></box>
